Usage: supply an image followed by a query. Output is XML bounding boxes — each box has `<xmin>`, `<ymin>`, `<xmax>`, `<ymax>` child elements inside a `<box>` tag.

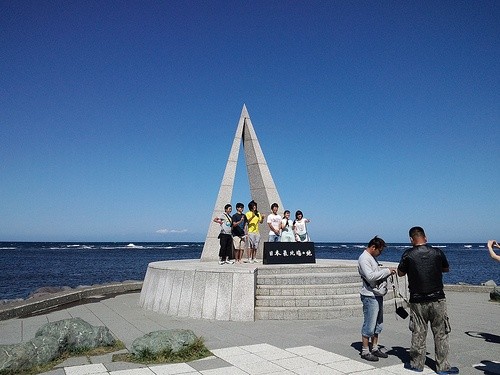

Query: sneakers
<box><xmin>373</xmin><ymin>349</ymin><xmax>388</xmax><ymax>358</ymax></box>
<box><xmin>437</xmin><ymin>366</ymin><xmax>460</xmax><ymax>375</ymax></box>
<box><xmin>362</xmin><ymin>353</ymin><xmax>379</xmax><ymax>361</ymax></box>
<box><xmin>404</xmin><ymin>362</ymin><xmax>424</xmax><ymax>372</ymax></box>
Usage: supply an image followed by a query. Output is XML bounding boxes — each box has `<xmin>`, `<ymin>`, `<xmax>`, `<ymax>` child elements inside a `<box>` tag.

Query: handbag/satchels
<box><xmin>397</xmin><ymin>306</ymin><xmax>408</xmax><ymax>319</ymax></box>
<box><xmin>374</xmin><ymin>278</ymin><xmax>389</xmax><ymax>297</ymax></box>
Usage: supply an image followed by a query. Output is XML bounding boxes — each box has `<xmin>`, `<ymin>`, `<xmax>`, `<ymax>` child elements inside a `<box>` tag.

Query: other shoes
<box><xmin>219</xmin><ymin>258</ymin><xmax>260</xmax><ymax>265</ymax></box>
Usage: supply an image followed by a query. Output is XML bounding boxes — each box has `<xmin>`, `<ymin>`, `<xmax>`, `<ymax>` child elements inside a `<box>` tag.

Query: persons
<box><xmin>293</xmin><ymin>210</ymin><xmax>311</xmax><ymax>242</ymax></box>
<box><xmin>232</xmin><ymin>202</ymin><xmax>248</xmax><ymax>264</ymax></box>
<box><xmin>396</xmin><ymin>226</ymin><xmax>459</xmax><ymax>375</ymax></box>
<box><xmin>213</xmin><ymin>204</ymin><xmax>234</xmax><ymax>264</ymax></box>
<box><xmin>280</xmin><ymin>210</ymin><xmax>296</xmax><ymax>242</ymax></box>
<box><xmin>245</xmin><ymin>200</ymin><xmax>265</xmax><ymax>264</ymax></box>
<box><xmin>487</xmin><ymin>239</ymin><xmax>500</xmax><ymax>261</ymax></box>
<box><xmin>267</xmin><ymin>203</ymin><xmax>282</xmax><ymax>241</ymax></box>
<box><xmin>357</xmin><ymin>235</ymin><xmax>396</xmax><ymax>361</ymax></box>
